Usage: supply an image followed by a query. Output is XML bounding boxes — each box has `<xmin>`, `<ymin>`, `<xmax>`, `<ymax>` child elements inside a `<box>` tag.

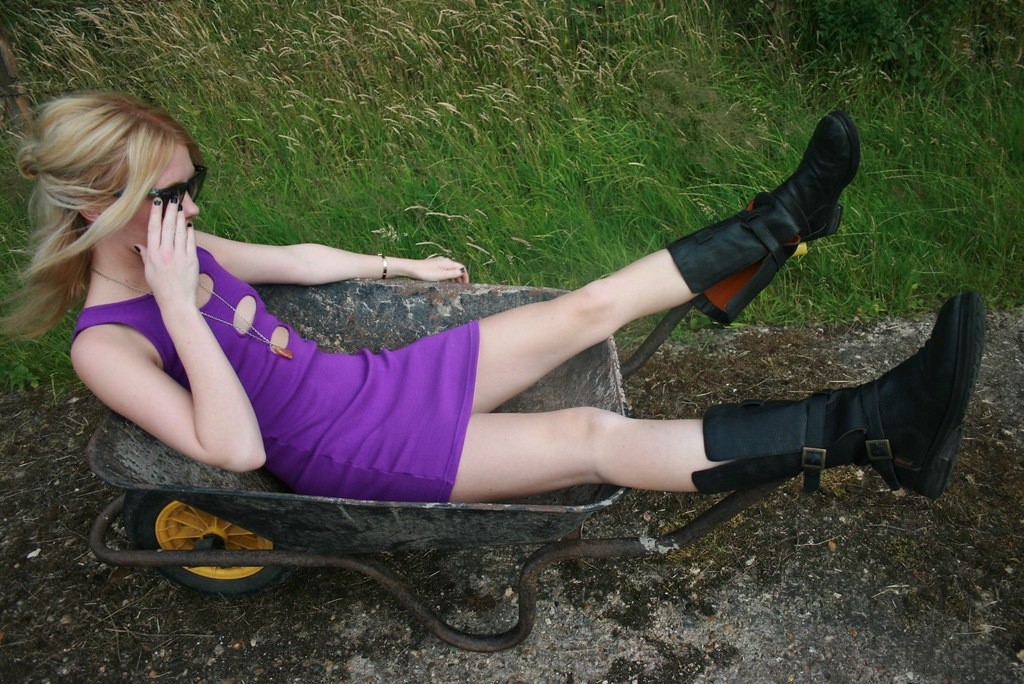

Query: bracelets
<box><xmin>379</xmin><ymin>254</ymin><xmax>389</xmax><ymax>279</ymax></box>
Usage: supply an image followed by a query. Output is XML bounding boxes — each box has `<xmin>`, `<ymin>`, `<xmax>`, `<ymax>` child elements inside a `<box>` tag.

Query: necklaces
<box><xmin>90</xmin><ymin>266</ymin><xmax>294</xmax><ymax>359</ymax></box>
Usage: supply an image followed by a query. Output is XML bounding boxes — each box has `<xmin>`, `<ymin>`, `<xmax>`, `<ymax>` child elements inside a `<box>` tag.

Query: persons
<box><xmin>2</xmin><ymin>94</ymin><xmax>984</xmax><ymax>496</ymax></box>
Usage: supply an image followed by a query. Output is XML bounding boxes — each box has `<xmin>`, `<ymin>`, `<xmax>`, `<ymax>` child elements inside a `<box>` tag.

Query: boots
<box><xmin>690</xmin><ymin>289</ymin><xmax>987</xmax><ymax>502</ymax></box>
<box><xmin>665</xmin><ymin>107</ymin><xmax>863</xmax><ymax>327</ymax></box>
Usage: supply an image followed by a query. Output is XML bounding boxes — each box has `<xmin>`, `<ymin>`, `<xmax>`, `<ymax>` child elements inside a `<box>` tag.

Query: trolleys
<box><xmin>85</xmin><ymin>242</ymin><xmax>811</xmax><ymax>654</ymax></box>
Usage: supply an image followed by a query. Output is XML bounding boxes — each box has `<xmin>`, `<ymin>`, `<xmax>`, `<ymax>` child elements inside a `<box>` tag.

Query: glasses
<box><xmin>108</xmin><ymin>163</ymin><xmax>209</xmax><ymax>217</ymax></box>
